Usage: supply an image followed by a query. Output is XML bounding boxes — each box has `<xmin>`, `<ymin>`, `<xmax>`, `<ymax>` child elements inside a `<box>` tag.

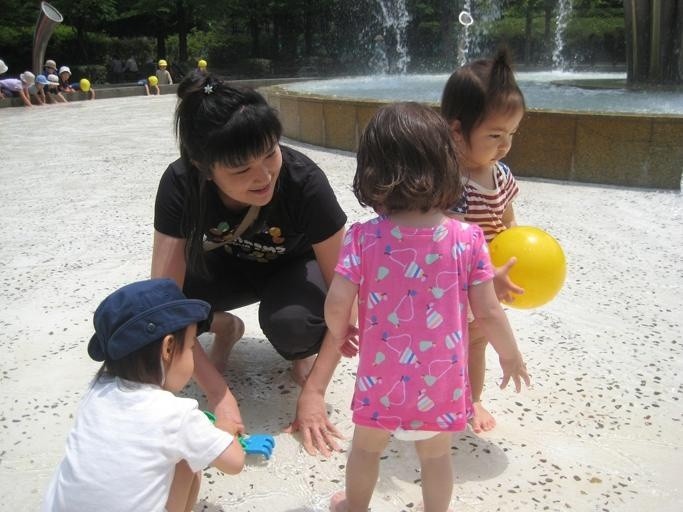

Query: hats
<box><xmin>0</xmin><ymin>60</ymin><xmax>8</xmax><ymax>73</ymax></box>
<box><xmin>44</xmin><ymin>60</ymin><xmax>58</xmax><ymax>71</ymax></box>
<box><xmin>48</xmin><ymin>74</ymin><xmax>59</xmax><ymax>86</ymax></box>
<box><xmin>36</xmin><ymin>75</ymin><xmax>48</xmax><ymax>84</ymax></box>
<box><xmin>59</xmin><ymin>66</ymin><xmax>72</xmax><ymax>75</ymax></box>
<box><xmin>20</xmin><ymin>71</ymin><xmax>35</xmax><ymax>86</ymax></box>
<box><xmin>88</xmin><ymin>279</ymin><xmax>214</xmax><ymax>361</ymax></box>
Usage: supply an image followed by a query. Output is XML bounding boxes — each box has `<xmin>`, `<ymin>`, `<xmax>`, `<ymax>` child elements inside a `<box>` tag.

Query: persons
<box><xmin>156</xmin><ymin>60</ymin><xmax>174</xmax><ymax>86</ymax></box>
<box><xmin>439</xmin><ymin>45</ymin><xmax>524</xmax><ymax>434</ymax></box>
<box><xmin>323</xmin><ymin>101</ymin><xmax>530</xmax><ymax>512</ymax></box>
<box><xmin>150</xmin><ymin>69</ymin><xmax>359</xmax><ymax>459</ymax></box>
<box><xmin>194</xmin><ymin>60</ymin><xmax>207</xmax><ymax>72</ymax></box>
<box><xmin>0</xmin><ymin>59</ymin><xmax>96</xmax><ymax>106</ymax></box>
<box><xmin>138</xmin><ymin>76</ymin><xmax>161</xmax><ymax>96</ymax></box>
<box><xmin>41</xmin><ymin>278</ymin><xmax>246</xmax><ymax>512</ymax></box>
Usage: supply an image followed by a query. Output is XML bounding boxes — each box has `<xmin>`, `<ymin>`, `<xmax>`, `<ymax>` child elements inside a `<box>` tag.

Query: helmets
<box><xmin>148</xmin><ymin>77</ymin><xmax>157</xmax><ymax>86</ymax></box>
<box><xmin>159</xmin><ymin>59</ymin><xmax>167</xmax><ymax>66</ymax></box>
<box><xmin>198</xmin><ymin>59</ymin><xmax>206</xmax><ymax>67</ymax></box>
<box><xmin>80</xmin><ymin>79</ymin><xmax>91</xmax><ymax>91</ymax></box>
